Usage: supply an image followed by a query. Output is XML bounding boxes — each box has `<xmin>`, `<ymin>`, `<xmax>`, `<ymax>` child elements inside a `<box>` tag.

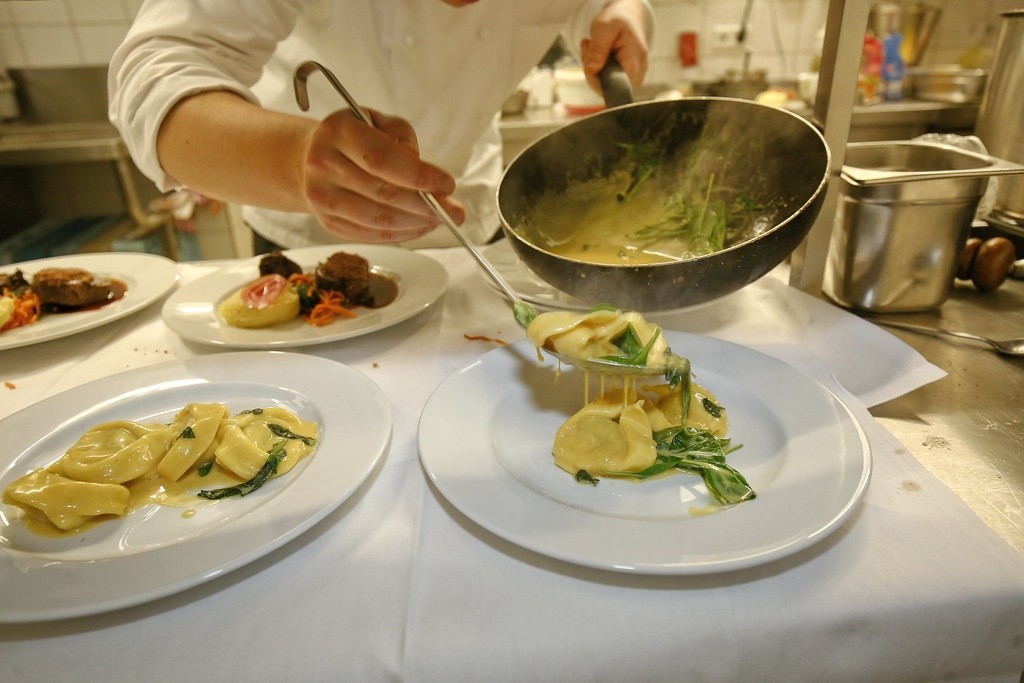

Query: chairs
<box><xmin>0</xmin><ymin>212</ymin><xmax>1024</xmax><ymax>683</ymax></box>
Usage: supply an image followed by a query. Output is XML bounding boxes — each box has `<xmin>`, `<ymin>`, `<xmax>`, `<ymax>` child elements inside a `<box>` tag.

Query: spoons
<box><xmin>864</xmin><ymin>316</ymin><xmax>1023</xmax><ymax>357</ymax></box>
<box><xmin>292</xmin><ymin>60</ymin><xmax>690</xmax><ymax>377</ymax></box>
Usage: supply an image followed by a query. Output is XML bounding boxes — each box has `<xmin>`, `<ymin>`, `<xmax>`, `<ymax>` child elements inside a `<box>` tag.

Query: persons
<box><xmin>106</xmin><ymin>0</ymin><xmax>656</xmax><ymax>259</ymax></box>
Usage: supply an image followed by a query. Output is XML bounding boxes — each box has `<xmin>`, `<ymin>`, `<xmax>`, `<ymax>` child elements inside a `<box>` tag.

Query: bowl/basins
<box><xmin>903</xmin><ymin>64</ymin><xmax>991</xmax><ymax>104</ymax></box>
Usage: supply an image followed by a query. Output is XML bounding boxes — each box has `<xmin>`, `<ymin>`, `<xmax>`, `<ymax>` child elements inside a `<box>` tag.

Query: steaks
<box><xmin>31</xmin><ymin>267</ymin><xmax>114</xmax><ymax>308</ymax></box>
<box><xmin>322</xmin><ymin>251</ymin><xmax>371</xmax><ymax>299</ymax></box>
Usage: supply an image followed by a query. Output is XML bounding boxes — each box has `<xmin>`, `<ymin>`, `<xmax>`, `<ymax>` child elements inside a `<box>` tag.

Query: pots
<box><xmin>496</xmin><ymin>51</ymin><xmax>831</xmax><ymax>315</ymax></box>
<box><xmin>974</xmin><ymin>8</ymin><xmax>1022</xmax><ymax>233</ymax></box>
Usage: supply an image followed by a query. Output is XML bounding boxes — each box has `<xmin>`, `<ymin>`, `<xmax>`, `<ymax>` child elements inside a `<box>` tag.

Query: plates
<box><xmin>419</xmin><ymin>327</ymin><xmax>874</xmax><ymax>576</ymax></box>
<box><xmin>2</xmin><ymin>350</ymin><xmax>391</xmax><ymax>626</ymax></box>
<box><xmin>0</xmin><ymin>253</ymin><xmax>183</xmax><ymax>351</ymax></box>
<box><xmin>160</xmin><ymin>242</ymin><xmax>450</xmax><ymax>348</ymax></box>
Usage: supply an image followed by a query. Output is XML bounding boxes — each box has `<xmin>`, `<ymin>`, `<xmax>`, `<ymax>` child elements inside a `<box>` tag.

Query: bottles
<box><xmin>856</xmin><ymin>28</ymin><xmax>883</xmax><ymax>105</ymax></box>
<box><xmin>879</xmin><ymin>28</ymin><xmax>905</xmax><ymax>102</ymax></box>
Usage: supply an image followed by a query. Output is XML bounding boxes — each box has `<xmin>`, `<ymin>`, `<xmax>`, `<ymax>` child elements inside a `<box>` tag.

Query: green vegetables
<box><xmin>167</xmin><ymin>402</ymin><xmax>315</xmax><ymax>499</ymax></box>
<box><xmin>516</xmin><ymin>301</ymin><xmax>758</xmax><ymax>504</ymax></box>
<box><xmin>620</xmin><ymin>135</ymin><xmax>771</xmax><ymax>261</ymax></box>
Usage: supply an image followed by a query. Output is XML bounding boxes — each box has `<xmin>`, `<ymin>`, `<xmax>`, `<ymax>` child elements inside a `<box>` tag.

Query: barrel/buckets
<box><xmin>822</xmin><ymin>140</ymin><xmax>1024</xmax><ymax>313</ymax></box>
